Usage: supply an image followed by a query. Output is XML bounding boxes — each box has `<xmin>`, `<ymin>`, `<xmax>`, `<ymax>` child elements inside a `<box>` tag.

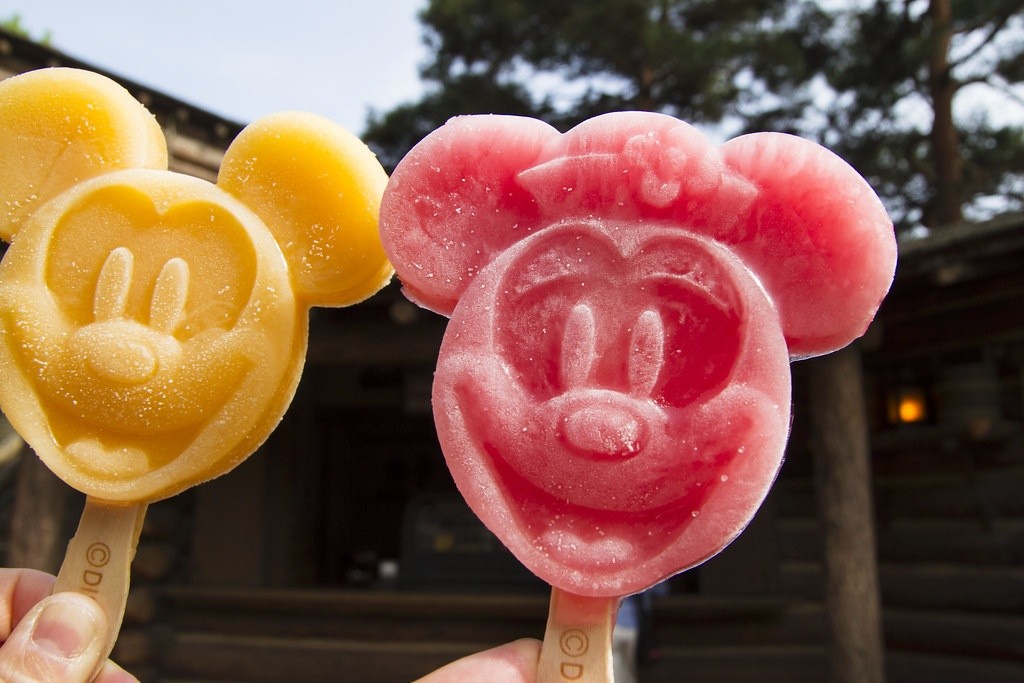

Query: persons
<box><xmin>413</xmin><ymin>637</ymin><xmax>544</xmax><ymax>683</ymax></box>
<box><xmin>0</xmin><ymin>568</ymin><xmax>142</xmax><ymax>682</ymax></box>
